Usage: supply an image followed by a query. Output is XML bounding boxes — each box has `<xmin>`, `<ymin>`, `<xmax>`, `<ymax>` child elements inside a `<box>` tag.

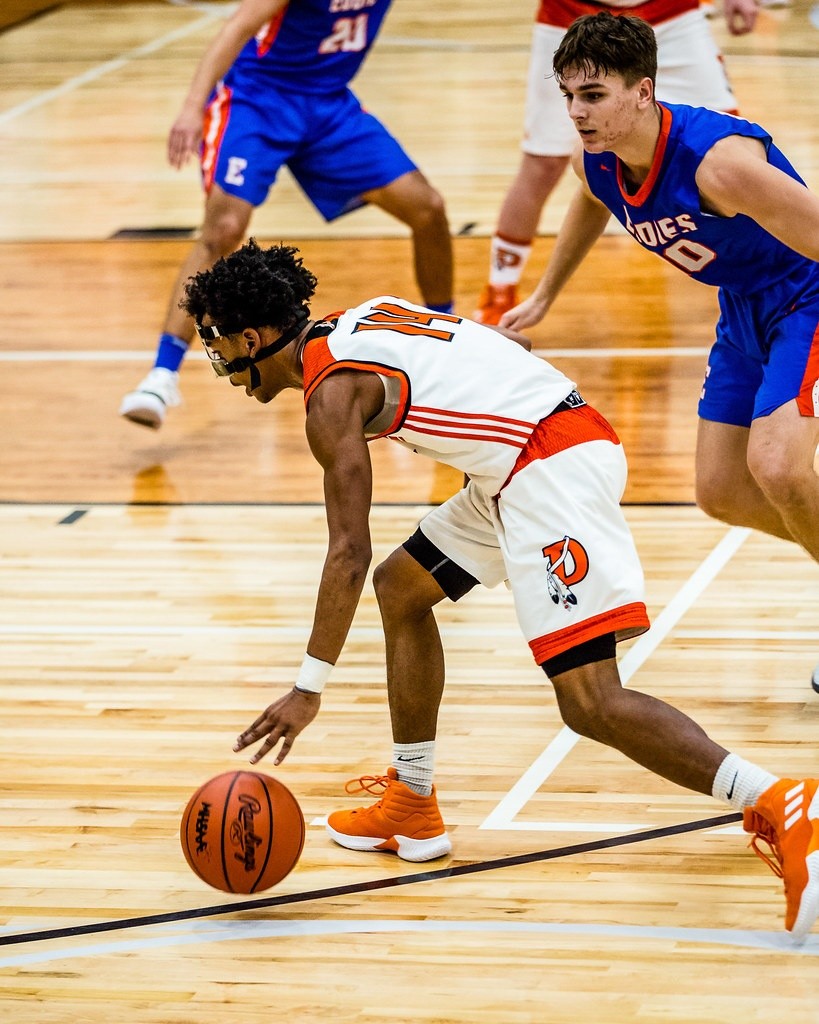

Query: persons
<box><xmin>474</xmin><ymin>0</ymin><xmax>759</xmax><ymax>326</ymax></box>
<box><xmin>498</xmin><ymin>12</ymin><xmax>819</xmax><ymax>693</ymax></box>
<box><xmin>181</xmin><ymin>239</ymin><xmax>819</xmax><ymax>940</ymax></box>
<box><xmin>120</xmin><ymin>0</ymin><xmax>455</xmax><ymax>429</ymax></box>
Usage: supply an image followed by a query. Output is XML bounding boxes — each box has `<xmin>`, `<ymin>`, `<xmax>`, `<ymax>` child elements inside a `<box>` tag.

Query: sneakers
<box><xmin>744</xmin><ymin>776</ymin><xmax>819</xmax><ymax>939</ymax></box>
<box><xmin>326</xmin><ymin>767</ymin><xmax>452</xmax><ymax>861</ymax></box>
<box><xmin>119</xmin><ymin>368</ymin><xmax>180</xmax><ymax>429</ymax></box>
<box><xmin>479</xmin><ymin>284</ymin><xmax>517</xmax><ymax>325</ymax></box>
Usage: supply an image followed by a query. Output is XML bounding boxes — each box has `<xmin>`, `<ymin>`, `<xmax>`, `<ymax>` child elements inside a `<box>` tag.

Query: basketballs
<box><xmin>178</xmin><ymin>770</ymin><xmax>307</xmax><ymax>895</ymax></box>
